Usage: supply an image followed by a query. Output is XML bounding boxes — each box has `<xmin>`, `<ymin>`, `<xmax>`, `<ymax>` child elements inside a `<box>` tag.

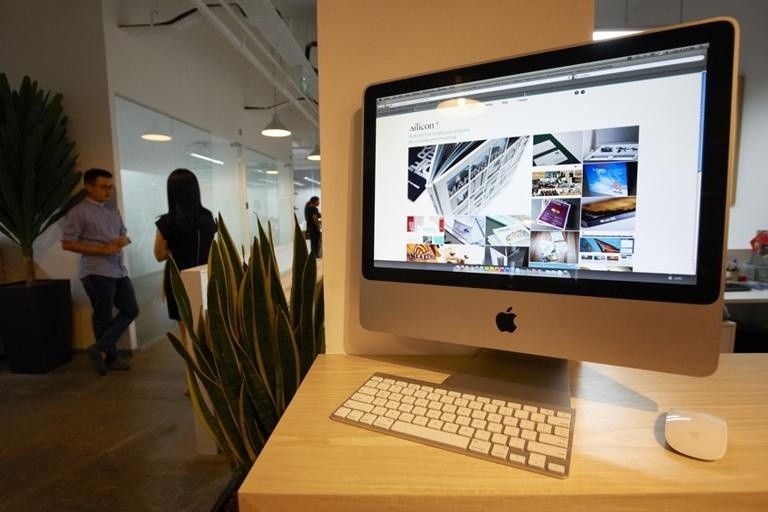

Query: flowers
<box><xmin>727</xmin><ymin>256</ymin><xmax>740</xmax><ymax>272</ymax></box>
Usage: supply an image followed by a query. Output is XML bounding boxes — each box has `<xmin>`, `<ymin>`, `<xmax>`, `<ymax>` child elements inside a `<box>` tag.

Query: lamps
<box><xmin>258</xmin><ymin>46</ymin><xmax>292</xmax><ymax>137</ymax></box>
<box><xmin>592</xmin><ymin>1</ymin><xmax>685</xmax><ymax>44</ymax></box>
<box><xmin>306</xmin><ymin>98</ymin><xmax>321</xmax><ymax>161</ymax></box>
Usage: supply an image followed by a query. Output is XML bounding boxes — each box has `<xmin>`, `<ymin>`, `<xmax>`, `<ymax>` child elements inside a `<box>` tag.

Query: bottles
<box><xmin>726</xmin><ymin>268</ymin><xmax>738</xmax><ymax>280</ymax></box>
<box><xmin>742</xmin><ymin>250</ymin><xmax>767</xmax><ymax>282</ymax></box>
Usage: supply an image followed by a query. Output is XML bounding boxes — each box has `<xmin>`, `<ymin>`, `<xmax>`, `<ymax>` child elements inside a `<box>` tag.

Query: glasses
<box><xmin>90</xmin><ymin>184</ymin><xmax>115</xmax><ymax>191</ymax></box>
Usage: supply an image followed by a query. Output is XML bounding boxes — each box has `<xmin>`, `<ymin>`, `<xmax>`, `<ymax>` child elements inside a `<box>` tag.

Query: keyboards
<box><xmin>330</xmin><ymin>372</ymin><xmax>576</xmax><ymax>480</ymax></box>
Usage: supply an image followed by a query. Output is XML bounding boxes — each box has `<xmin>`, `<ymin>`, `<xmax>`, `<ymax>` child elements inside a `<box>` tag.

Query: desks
<box><xmin>235</xmin><ymin>352</ymin><xmax>768</xmax><ymax>511</ymax></box>
<box><xmin>720</xmin><ymin>279</ymin><xmax>768</xmax><ymax>353</ymax></box>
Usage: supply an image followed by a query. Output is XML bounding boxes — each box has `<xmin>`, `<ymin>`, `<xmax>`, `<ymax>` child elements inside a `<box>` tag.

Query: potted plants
<box><xmin>0</xmin><ymin>70</ymin><xmax>89</xmax><ymax>376</ymax></box>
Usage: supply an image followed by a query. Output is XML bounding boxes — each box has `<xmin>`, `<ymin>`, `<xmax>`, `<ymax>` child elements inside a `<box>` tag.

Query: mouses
<box><xmin>665</xmin><ymin>410</ymin><xmax>728</xmax><ymax>462</ymax></box>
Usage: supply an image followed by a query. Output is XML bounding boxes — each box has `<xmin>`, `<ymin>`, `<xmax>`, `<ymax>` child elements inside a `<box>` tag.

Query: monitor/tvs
<box><xmin>358</xmin><ymin>15</ymin><xmax>740</xmax><ymax>408</ymax></box>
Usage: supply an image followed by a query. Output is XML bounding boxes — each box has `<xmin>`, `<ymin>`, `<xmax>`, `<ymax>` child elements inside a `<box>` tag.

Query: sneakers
<box><xmin>86</xmin><ymin>343</ymin><xmax>109</xmax><ymax>376</ymax></box>
<box><xmin>107</xmin><ymin>358</ymin><xmax>130</xmax><ymax>371</ymax></box>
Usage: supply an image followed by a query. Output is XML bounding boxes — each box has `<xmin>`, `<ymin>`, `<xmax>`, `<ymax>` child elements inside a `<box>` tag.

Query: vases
<box><xmin>725</xmin><ymin>270</ymin><xmax>739</xmax><ymax>282</ymax></box>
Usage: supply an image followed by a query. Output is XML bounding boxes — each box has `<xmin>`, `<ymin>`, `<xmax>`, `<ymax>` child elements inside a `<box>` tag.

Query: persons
<box><xmin>57</xmin><ymin>167</ymin><xmax>141</xmax><ymax>376</ymax></box>
<box><xmin>304</xmin><ymin>195</ymin><xmax>324</xmax><ymax>257</ymax></box>
<box><xmin>153</xmin><ymin>166</ymin><xmax>217</xmax><ymax>398</ymax></box>
